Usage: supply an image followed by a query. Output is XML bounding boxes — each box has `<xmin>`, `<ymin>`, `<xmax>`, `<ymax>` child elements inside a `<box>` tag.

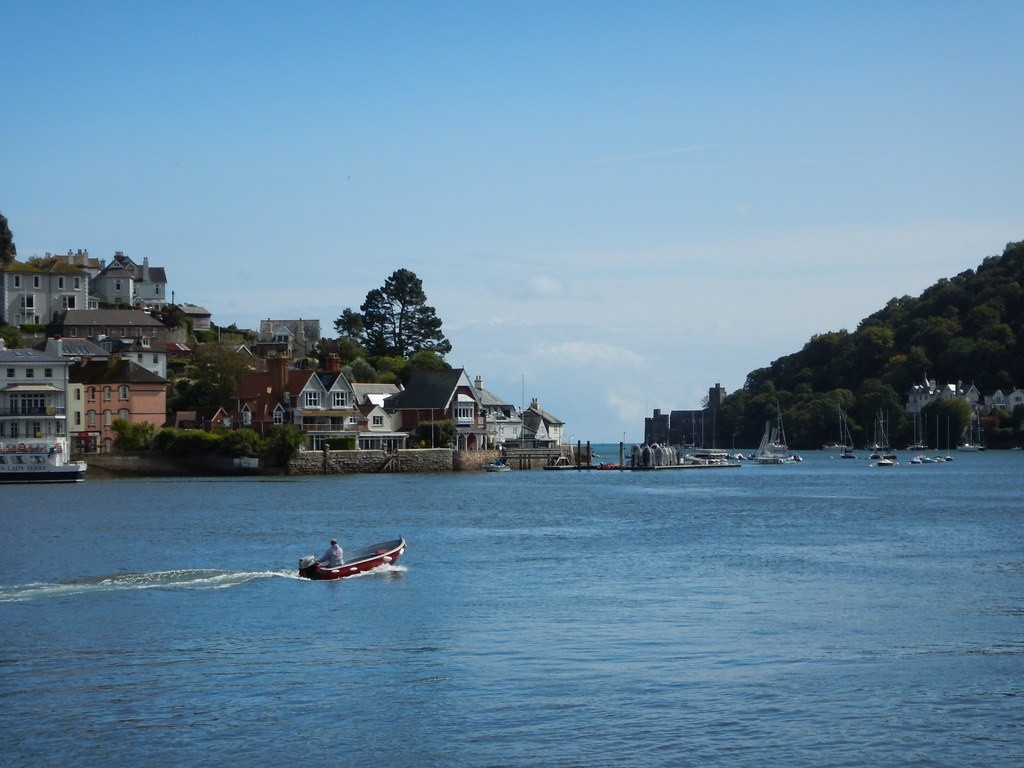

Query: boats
<box><xmin>957</xmin><ymin>445</ymin><xmax>979</xmax><ymax>453</ymax></box>
<box><xmin>298</xmin><ymin>536</ymin><xmax>408</xmax><ymax>582</ymax></box>
<box><xmin>822</xmin><ymin>445</ymin><xmax>847</xmax><ymax>452</ymax></box>
<box><xmin>978</xmin><ymin>445</ymin><xmax>985</xmax><ymax>451</ymax></box>
<box><xmin>481</xmin><ymin>460</ymin><xmax>512</xmax><ymax>473</ymax></box>
<box><xmin>879</xmin><ymin>459</ymin><xmax>894</xmax><ymax>466</ymax></box>
<box><xmin>841</xmin><ymin>453</ymin><xmax>855</xmax><ymax>459</ymax></box>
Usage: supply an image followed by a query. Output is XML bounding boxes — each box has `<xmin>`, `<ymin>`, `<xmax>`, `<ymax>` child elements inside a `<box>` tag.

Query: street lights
<box><xmin>569</xmin><ymin>435</ymin><xmax>575</xmax><ymax>463</ymax></box>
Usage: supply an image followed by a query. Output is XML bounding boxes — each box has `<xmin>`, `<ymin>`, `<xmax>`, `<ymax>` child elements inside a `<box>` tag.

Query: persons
<box><xmin>495</xmin><ymin>459</ymin><xmax>502</xmax><ymax>466</ymax></box>
<box><xmin>316</xmin><ymin>539</ymin><xmax>344</xmax><ymax>568</ymax></box>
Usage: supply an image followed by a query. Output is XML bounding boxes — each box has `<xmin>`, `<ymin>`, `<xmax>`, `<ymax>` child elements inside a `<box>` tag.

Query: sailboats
<box><xmin>876</xmin><ymin>409</ymin><xmax>898</xmax><ymax>458</ymax></box>
<box><xmin>908</xmin><ymin>409</ymin><xmax>954</xmax><ymax>464</ymax></box>
<box><xmin>745</xmin><ymin>399</ymin><xmax>804</xmax><ymax>464</ymax></box>
<box><xmin>870</xmin><ymin>418</ymin><xmax>880</xmax><ymax>459</ymax></box>
